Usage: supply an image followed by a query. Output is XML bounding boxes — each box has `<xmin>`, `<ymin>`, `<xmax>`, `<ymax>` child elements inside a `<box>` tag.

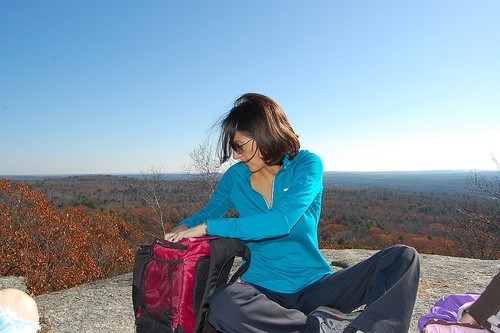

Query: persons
<box><xmin>163</xmin><ymin>93</ymin><xmax>420</xmax><ymax>333</ymax></box>
<box><xmin>460</xmin><ymin>273</ymin><xmax>500</xmax><ymax>325</ymax></box>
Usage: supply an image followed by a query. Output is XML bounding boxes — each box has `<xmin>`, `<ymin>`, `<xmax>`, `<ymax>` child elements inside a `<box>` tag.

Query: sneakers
<box><xmin>309</xmin><ymin>306</ymin><xmax>362</xmax><ymax>333</ymax></box>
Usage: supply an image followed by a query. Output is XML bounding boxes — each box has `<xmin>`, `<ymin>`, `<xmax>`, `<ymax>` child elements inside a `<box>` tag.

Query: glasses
<box><xmin>232</xmin><ymin>136</ymin><xmax>257</xmax><ymax>154</ymax></box>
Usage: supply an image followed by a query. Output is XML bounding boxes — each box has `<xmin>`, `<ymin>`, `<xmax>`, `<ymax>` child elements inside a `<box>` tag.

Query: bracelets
<box><xmin>205</xmin><ymin>222</ymin><xmax>208</xmax><ymax>234</ymax></box>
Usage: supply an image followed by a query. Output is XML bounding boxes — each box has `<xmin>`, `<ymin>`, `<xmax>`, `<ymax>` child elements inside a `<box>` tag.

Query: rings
<box><xmin>169</xmin><ymin>236</ymin><xmax>174</xmax><ymax>240</ymax></box>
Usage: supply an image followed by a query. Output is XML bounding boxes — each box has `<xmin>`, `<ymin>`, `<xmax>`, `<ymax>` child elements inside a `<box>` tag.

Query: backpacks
<box><xmin>132</xmin><ymin>230</ymin><xmax>251</xmax><ymax>333</ymax></box>
